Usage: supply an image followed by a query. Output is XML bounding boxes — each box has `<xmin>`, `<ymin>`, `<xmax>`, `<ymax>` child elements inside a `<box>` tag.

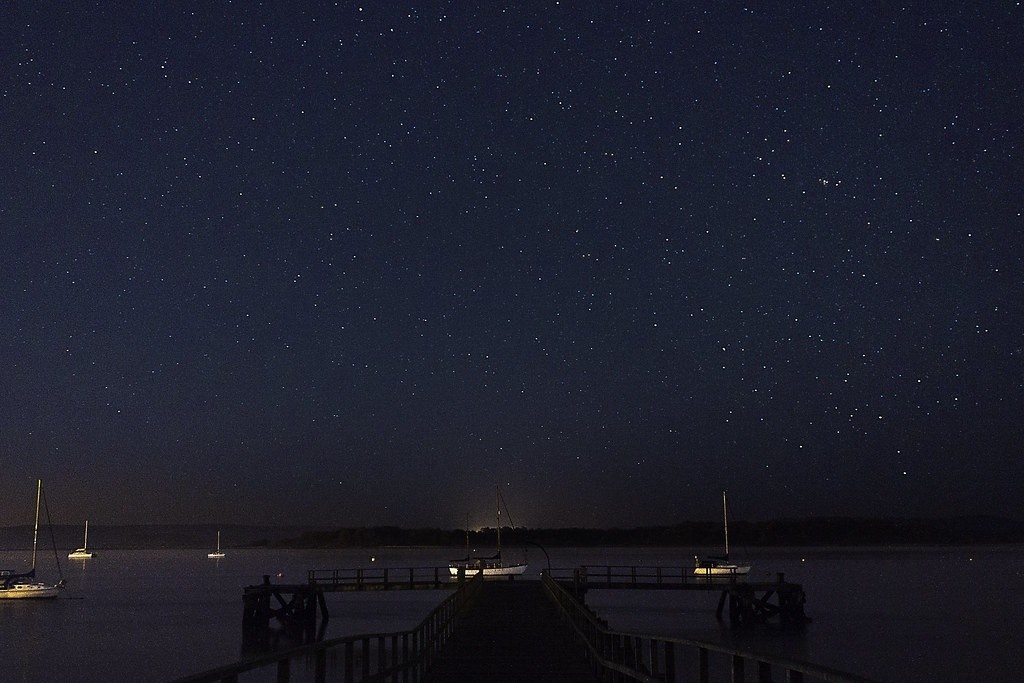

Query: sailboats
<box><xmin>0</xmin><ymin>479</ymin><xmax>67</xmax><ymax>600</ymax></box>
<box><xmin>694</xmin><ymin>492</ymin><xmax>753</xmax><ymax>575</ymax></box>
<box><xmin>448</xmin><ymin>486</ymin><xmax>528</xmax><ymax>577</ymax></box>
<box><xmin>68</xmin><ymin>520</ymin><xmax>97</xmax><ymax>558</ymax></box>
<box><xmin>208</xmin><ymin>531</ymin><xmax>226</xmax><ymax>557</ymax></box>
